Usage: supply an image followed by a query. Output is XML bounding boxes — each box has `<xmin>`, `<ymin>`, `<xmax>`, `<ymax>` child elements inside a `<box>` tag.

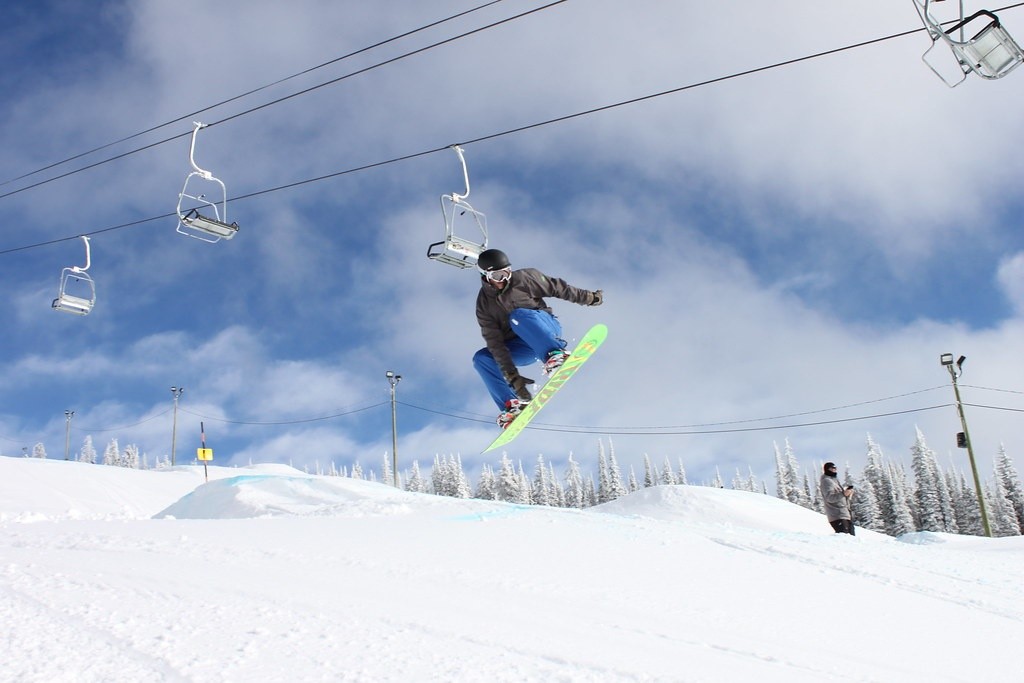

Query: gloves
<box><xmin>512</xmin><ymin>377</ymin><xmax>535</xmax><ymax>401</ymax></box>
<box><xmin>592</xmin><ymin>289</ymin><xmax>603</xmax><ymax>306</ymax></box>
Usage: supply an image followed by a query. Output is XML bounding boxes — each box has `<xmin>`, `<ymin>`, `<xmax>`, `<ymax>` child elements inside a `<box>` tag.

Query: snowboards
<box><xmin>478</xmin><ymin>322</ymin><xmax>610</xmax><ymax>455</ymax></box>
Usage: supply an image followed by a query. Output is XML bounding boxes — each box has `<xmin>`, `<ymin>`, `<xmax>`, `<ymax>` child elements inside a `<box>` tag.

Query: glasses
<box><xmin>487</xmin><ymin>266</ymin><xmax>512</xmax><ymax>282</ymax></box>
<box><xmin>832</xmin><ymin>466</ymin><xmax>837</xmax><ymax>470</ymax></box>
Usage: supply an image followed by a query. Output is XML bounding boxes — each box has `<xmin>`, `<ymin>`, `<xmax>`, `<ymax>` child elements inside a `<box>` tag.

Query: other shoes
<box><xmin>545</xmin><ymin>352</ymin><xmax>568</xmax><ymax>371</ymax></box>
<box><xmin>497</xmin><ymin>408</ymin><xmax>521</xmax><ymax>427</ymax></box>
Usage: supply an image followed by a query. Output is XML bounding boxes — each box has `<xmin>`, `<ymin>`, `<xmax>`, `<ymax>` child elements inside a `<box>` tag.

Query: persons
<box><xmin>470</xmin><ymin>247</ymin><xmax>604</xmax><ymax>429</ymax></box>
<box><xmin>819</xmin><ymin>461</ymin><xmax>857</xmax><ymax>539</ymax></box>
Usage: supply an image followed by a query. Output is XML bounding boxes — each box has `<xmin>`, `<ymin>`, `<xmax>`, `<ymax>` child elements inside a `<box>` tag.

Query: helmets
<box><xmin>478</xmin><ymin>248</ymin><xmax>511</xmax><ymax>271</ymax></box>
<box><xmin>824</xmin><ymin>462</ymin><xmax>834</xmax><ymax>471</ymax></box>
